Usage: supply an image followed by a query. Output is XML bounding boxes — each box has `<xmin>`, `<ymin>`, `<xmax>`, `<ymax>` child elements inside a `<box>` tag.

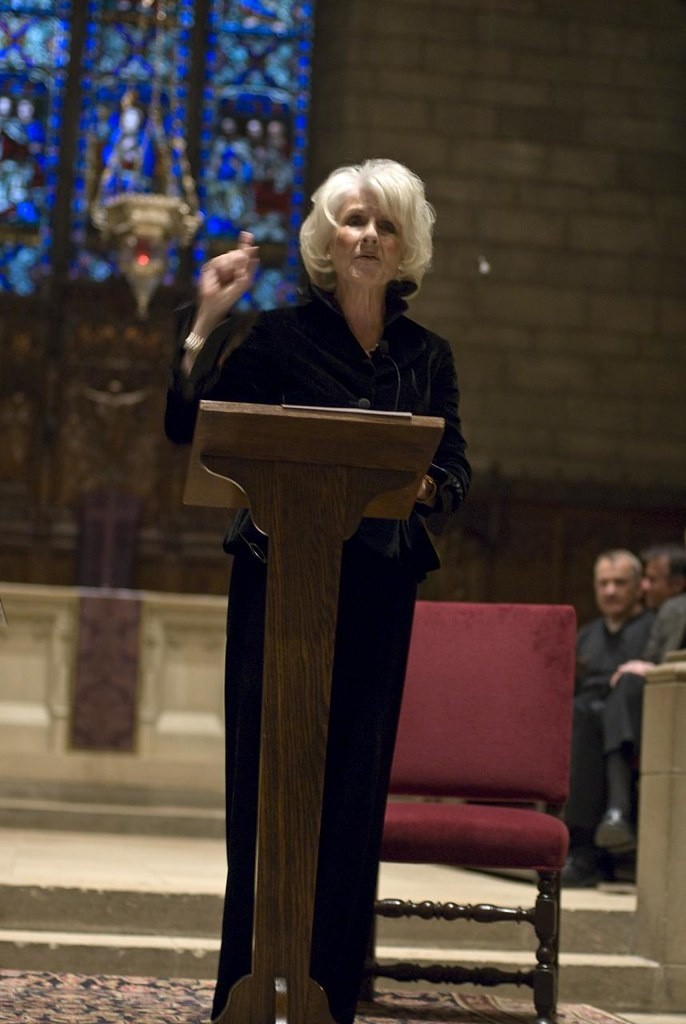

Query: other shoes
<box><xmin>561</xmin><ymin>847</ymin><xmax>598</xmax><ymax>888</ymax></box>
<box><xmin>594</xmin><ymin>809</ymin><xmax>637</xmax><ymax>853</ymax></box>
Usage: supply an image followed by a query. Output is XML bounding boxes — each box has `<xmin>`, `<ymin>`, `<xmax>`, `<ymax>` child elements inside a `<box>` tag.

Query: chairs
<box><xmin>361</xmin><ymin>601</ymin><xmax>576</xmax><ymax>1020</ymax></box>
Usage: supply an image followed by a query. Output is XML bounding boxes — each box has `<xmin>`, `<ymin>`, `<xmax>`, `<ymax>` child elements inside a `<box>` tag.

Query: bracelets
<box><xmin>184</xmin><ymin>334</ymin><xmax>205</xmax><ymax>354</ymax></box>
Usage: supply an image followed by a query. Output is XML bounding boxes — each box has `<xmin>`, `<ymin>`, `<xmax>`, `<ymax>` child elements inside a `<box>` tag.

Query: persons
<box><xmin>157</xmin><ymin>158</ymin><xmax>472</xmax><ymax>1024</ymax></box>
<box><xmin>560</xmin><ymin>545</ymin><xmax>686</xmax><ymax>889</ymax></box>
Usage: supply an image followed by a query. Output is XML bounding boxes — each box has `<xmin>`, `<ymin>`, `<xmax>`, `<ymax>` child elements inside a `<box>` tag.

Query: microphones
<box><xmin>398</xmin><ymin>263</ymin><xmax>404</xmax><ymax>270</ymax></box>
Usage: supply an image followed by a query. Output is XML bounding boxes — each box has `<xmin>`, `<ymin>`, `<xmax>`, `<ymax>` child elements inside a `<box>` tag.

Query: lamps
<box><xmin>91</xmin><ymin>0</ymin><xmax>199</xmax><ymax>320</ymax></box>
